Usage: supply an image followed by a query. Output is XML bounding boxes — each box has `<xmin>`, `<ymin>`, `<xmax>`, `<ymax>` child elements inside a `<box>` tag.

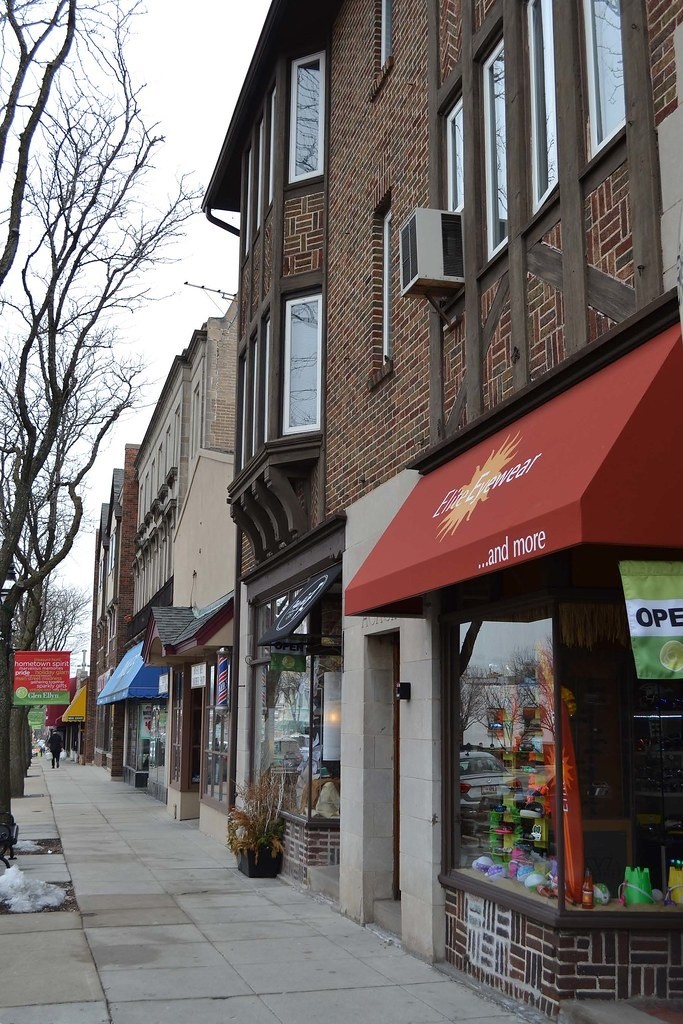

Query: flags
<box><xmin>616</xmin><ymin>560</ymin><xmax>683</xmax><ymax>680</ymax></box>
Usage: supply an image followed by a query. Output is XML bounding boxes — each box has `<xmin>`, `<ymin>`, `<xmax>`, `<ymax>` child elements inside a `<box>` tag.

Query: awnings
<box><xmin>345</xmin><ymin>318</ymin><xmax>683</xmax><ymax>618</ymax></box>
<box><xmin>256</xmin><ymin>562</ymin><xmax>342</xmax><ymax>645</ymax></box>
<box><xmin>60</xmin><ymin>685</ymin><xmax>87</xmax><ymax>723</ymax></box>
<box><xmin>96</xmin><ymin>639</ymin><xmax>168</xmax><ymax>706</ymax></box>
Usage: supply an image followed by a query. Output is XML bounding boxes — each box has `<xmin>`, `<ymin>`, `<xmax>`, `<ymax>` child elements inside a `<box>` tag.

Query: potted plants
<box><xmin>228</xmin><ymin>763</ymin><xmax>289</xmax><ymax>878</ymax></box>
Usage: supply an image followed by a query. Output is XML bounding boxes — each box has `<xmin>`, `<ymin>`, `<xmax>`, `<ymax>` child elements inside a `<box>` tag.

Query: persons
<box><xmin>47</xmin><ymin>730</ymin><xmax>62</xmax><ymax>769</ymax></box>
<box><xmin>31</xmin><ymin>736</ymin><xmax>48</xmax><ymax>757</ymax></box>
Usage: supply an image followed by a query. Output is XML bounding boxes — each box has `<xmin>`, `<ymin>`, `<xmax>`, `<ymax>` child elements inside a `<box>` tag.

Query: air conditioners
<box><xmin>398</xmin><ymin>208</ymin><xmax>465</xmax><ymax>298</ymax></box>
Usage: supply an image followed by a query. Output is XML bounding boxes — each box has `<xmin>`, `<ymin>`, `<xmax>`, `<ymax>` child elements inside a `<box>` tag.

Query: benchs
<box><xmin>0</xmin><ymin>811</ymin><xmax>19</xmax><ymax>868</ymax></box>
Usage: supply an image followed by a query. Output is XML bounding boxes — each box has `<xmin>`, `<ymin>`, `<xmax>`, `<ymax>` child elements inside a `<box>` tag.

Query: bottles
<box><xmin>581</xmin><ymin>865</ymin><xmax>594</xmax><ymax>909</ymax></box>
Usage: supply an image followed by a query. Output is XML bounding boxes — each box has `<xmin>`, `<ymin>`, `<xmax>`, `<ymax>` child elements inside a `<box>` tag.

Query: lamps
<box><xmin>323</xmin><ymin>672</ymin><xmax>341</xmax><ymax>761</ymax></box>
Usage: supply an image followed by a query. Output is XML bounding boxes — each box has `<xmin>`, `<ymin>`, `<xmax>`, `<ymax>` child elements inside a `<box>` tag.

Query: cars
<box><xmin>292</xmin><ymin>734</ymin><xmax>309</xmax><ymax>761</ymax></box>
<box><xmin>269</xmin><ymin>739</ymin><xmax>304</xmax><ymax>774</ymax></box>
<box><xmin>460</xmin><ymin>752</ymin><xmax>522</xmax><ymax>818</ymax></box>
